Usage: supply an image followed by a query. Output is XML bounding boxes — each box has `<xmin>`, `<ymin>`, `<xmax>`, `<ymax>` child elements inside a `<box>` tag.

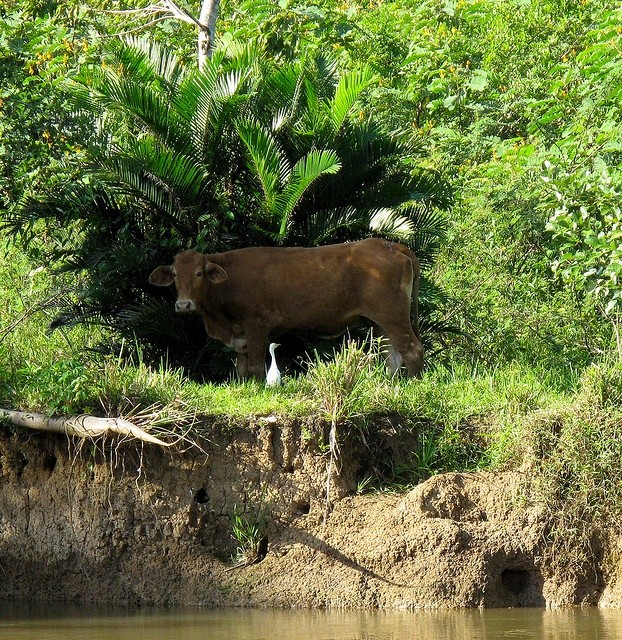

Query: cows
<box><xmin>147</xmin><ymin>236</ymin><xmax>425</xmax><ymax>386</ymax></box>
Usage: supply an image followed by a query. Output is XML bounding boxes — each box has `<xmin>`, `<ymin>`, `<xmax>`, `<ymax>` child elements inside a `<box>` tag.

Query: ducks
<box><xmin>265</xmin><ymin>342</ymin><xmax>283</xmax><ymax>387</ymax></box>
